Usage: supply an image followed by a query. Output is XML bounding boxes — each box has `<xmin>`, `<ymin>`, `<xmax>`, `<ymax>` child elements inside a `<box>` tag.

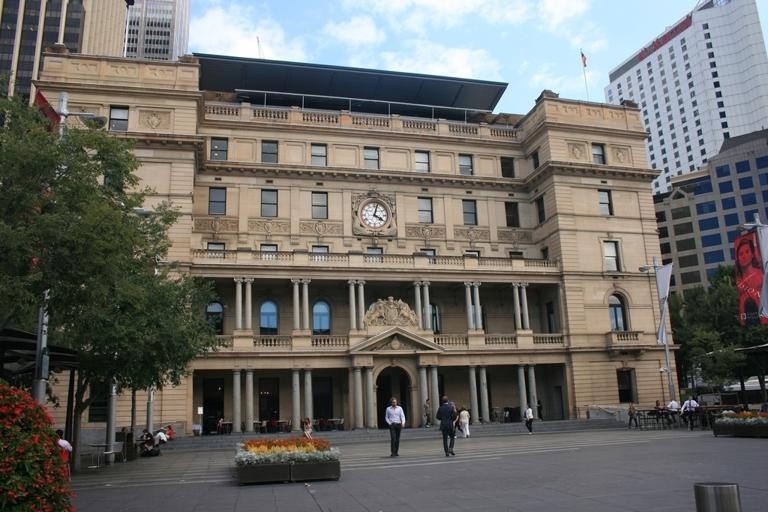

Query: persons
<box><xmin>55</xmin><ymin>430</ymin><xmax>72</xmax><ymax>452</ymax></box>
<box><xmin>627</xmin><ymin>395</ymin><xmax>707</xmax><ymax>430</ymax></box>
<box><xmin>385</xmin><ymin>397</ymin><xmax>406</xmax><ymax>457</ymax></box>
<box><xmin>423</xmin><ymin>395</ymin><xmax>470</xmax><ymax>457</ymax></box>
<box><xmin>118</xmin><ymin>424</ymin><xmax>175</xmax><ymax>462</ymax></box>
<box><xmin>732</xmin><ymin>238</ymin><xmax>768</xmax><ymax>327</ymax></box>
<box><xmin>525</xmin><ymin>399</ymin><xmax>544</xmax><ymax>434</ymax></box>
<box><xmin>383</xmin><ymin>295</ymin><xmax>403</xmax><ymax>325</ymax></box>
<box><xmin>304</xmin><ymin>418</ymin><xmax>313</xmax><ymax>439</ymax></box>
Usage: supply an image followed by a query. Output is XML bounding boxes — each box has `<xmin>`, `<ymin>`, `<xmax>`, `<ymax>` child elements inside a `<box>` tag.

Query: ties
<box><xmin>689</xmin><ymin>400</ymin><xmax>691</xmax><ymax>411</ymax></box>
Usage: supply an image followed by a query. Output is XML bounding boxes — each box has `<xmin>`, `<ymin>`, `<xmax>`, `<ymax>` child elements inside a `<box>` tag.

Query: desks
<box><xmin>634</xmin><ymin>403</ymin><xmax>745</xmax><ymax>431</ymax></box>
<box><xmin>301</xmin><ymin>418</ymin><xmax>342</xmax><ymax>432</ymax></box>
<box><xmin>221</xmin><ymin>421</ymin><xmax>233</xmax><ymax>433</ymax></box>
<box><xmin>240</xmin><ymin>419</ymin><xmax>287</xmax><ymax>433</ymax></box>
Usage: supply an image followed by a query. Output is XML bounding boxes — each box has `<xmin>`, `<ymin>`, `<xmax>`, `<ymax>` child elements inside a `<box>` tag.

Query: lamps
<box><xmin>197</xmin><ymin>406</ymin><xmax>204</xmax><ymax>435</ymax></box>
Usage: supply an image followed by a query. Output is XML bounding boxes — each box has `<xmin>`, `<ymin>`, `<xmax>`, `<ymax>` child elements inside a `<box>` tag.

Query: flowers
<box><xmin>234</xmin><ymin>434</ymin><xmax>342</xmax><ymax>466</ymax></box>
<box><xmin>713</xmin><ymin>408</ymin><xmax>768</xmax><ymax>426</ymax></box>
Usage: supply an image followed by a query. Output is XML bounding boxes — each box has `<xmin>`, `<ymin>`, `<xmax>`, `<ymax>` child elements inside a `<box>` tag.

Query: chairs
<box><xmin>59</xmin><ymin>434</ymin><xmax>157</xmax><ymax>482</ymax></box>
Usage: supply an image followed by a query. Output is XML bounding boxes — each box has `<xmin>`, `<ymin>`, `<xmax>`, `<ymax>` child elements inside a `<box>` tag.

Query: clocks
<box><xmin>357</xmin><ymin>198</ymin><xmax>393</xmax><ymax>231</ymax></box>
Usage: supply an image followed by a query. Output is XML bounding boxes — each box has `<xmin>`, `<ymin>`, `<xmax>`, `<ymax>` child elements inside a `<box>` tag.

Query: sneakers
<box><xmin>448</xmin><ymin>435</ymin><xmax>457</xmax><ymax>438</ymax></box>
<box><xmin>466</xmin><ymin>435</ymin><xmax>468</xmax><ymax>438</ymax></box>
<box><xmin>426</xmin><ymin>425</ymin><xmax>431</xmax><ymax>427</ymax></box>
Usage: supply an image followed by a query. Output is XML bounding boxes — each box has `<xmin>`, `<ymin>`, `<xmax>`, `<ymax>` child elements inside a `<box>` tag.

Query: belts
<box><xmin>392</xmin><ymin>423</ymin><xmax>401</xmax><ymax>425</ymax></box>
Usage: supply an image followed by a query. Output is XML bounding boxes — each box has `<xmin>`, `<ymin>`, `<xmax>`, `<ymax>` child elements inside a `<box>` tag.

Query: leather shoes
<box><xmin>446</xmin><ymin>448</ymin><xmax>455</xmax><ymax>456</ymax></box>
<box><xmin>391</xmin><ymin>453</ymin><xmax>398</xmax><ymax>456</ymax></box>
